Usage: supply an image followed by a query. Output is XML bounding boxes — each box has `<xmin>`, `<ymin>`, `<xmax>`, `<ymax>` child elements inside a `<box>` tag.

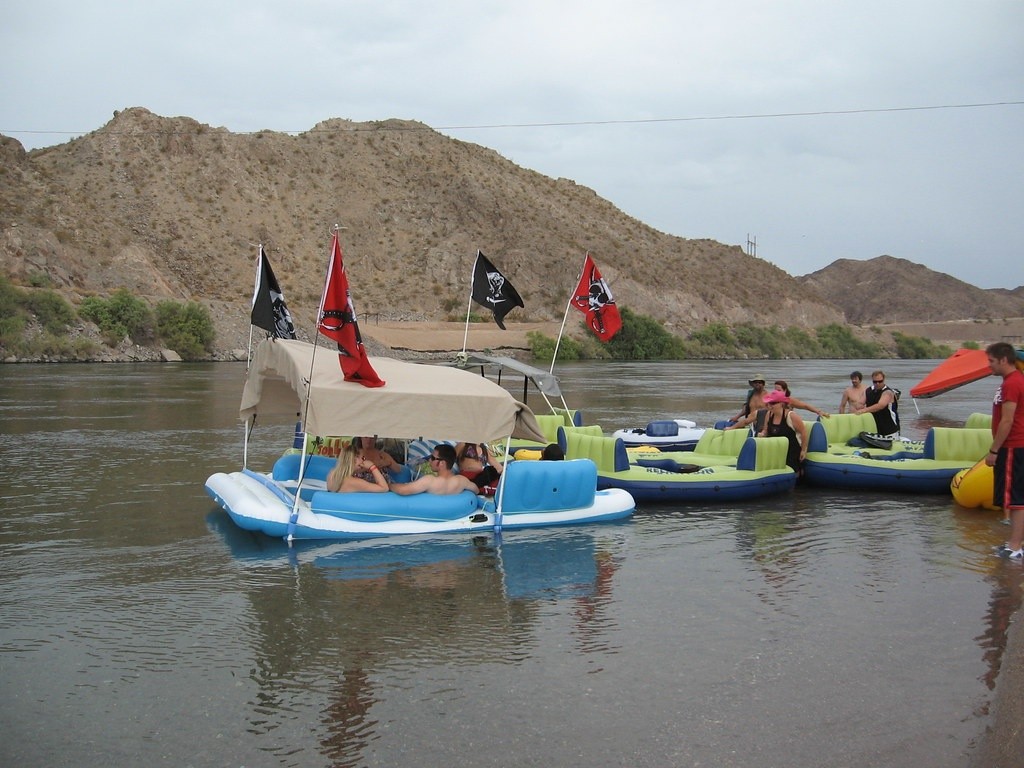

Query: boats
<box><xmin>205</xmin><ymin>451</ymin><xmax>635</xmax><ymax>541</ymax></box>
<box><xmin>799</xmin><ymin>412</ymin><xmax>992</xmax><ymax>496</ymax></box>
<box><xmin>301</xmin><ymin>351</ymin><xmax>797</xmax><ymax>506</ymax></box>
<box><xmin>610</xmin><ymin>419</ymin><xmax>705</xmax><ymax>451</ymax></box>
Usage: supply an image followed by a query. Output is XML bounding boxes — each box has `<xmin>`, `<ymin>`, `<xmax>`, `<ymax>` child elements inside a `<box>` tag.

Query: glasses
<box><xmin>769</xmin><ymin>402</ymin><xmax>780</xmax><ymax>405</ymax></box>
<box><xmin>873</xmin><ymin>380</ymin><xmax>883</xmax><ymax>383</ymax></box>
<box><xmin>356</xmin><ymin>455</ymin><xmax>366</xmax><ymax>461</ymax></box>
<box><xmin>754</xmin><ymin>382</ymin><xmax>763</xmax><ymax>384</ymax></box>
<box><xmin>431</xmin><ymin>454</ymin><xmax>446</xmax><ymax>461</ymax></box>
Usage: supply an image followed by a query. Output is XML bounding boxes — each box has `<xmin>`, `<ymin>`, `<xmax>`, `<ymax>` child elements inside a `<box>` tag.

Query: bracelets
<box><xmin>369</xmin><ymin>464</ymin><xmax>375</xmax><ymax>470</ymax></box>
<box><xmin>990</xmin><ymin>450</ymin><xmax>998</xmax><ymax>455</ymax></box>
<box><xmin>371</xmin><ymin>466</ymin><xmax>377</xmax><ymax>472</ymax></box>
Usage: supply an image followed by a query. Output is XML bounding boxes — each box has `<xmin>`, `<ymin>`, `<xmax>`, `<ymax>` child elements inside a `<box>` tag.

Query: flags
<box><xmin>568</xmin><ymin>256</ymin><xmax>622</xmax><ymax>340</ymax></box>
<box><xmin>469</xmin><ymin>252</ymin><xmax>524</xmax><ymax>329</ymax></box>
<box><xmin>250</xmin><ymin>249</ymin><xmax>299</xmax><ymax>341</ymax></box>
<box><xmin>316</xmin><ymin>239</ymin><xmax>386</xmax><ymax>388</ymax></box>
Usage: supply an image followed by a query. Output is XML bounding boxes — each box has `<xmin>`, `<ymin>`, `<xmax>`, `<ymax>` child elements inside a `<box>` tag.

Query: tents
<box><xmin>907</xmin><ymin>348</ymin><xmax>994</xmax><ymax>415</ymax></box>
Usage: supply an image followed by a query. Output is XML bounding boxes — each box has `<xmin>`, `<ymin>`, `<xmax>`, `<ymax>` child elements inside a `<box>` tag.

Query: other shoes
<box><xmin>988</xmin><ymin>541</ymin><xmax>1024</xmax><ymax>560</ymax></box>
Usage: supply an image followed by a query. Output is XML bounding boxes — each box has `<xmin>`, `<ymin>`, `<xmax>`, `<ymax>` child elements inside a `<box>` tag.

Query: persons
<box><xmin>839</xmin><ymin>370</ymin><xmax>869</xmax><ymax>414</ymax></box>
<box><xmin>984</xmin><ymin>342</ymin><xmax>1024</xmax><ymax>523</ymax></box>
<box><xmin>539</xmin><ymin>443</ymin><xmax>564</xmax><ymax>460</ymax></box>
<box><xmin>856</xmin><ymin>369</ymin><xmax>900</xmax><ymax>437</ymax></box>
<box><xmin>725</xmin><ymin>373</ymin><xmax>828</xmax><ymax>476</ymax></box>
<box><xmin>327</xmin><ymin>437</ymin><xmax>504</xmax><ymax>497</ymax></box>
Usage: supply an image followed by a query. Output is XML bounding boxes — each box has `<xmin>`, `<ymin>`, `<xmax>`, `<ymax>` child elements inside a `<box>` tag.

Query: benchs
<box><xmin>272</xmin><ymin>402</ymin><xmax>994</xmax><ymax>524</ymax></box>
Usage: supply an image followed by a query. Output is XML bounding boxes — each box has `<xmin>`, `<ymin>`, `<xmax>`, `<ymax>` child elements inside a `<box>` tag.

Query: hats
<box><xmin>748</xmin><ymin>374</ymin><xmax>766</xmax><ymax>386</ymax></box>
<box><xmin>761</xmin><ymin>390</ymin><xmax>790</xmax><ymax>403</ymax></box>
